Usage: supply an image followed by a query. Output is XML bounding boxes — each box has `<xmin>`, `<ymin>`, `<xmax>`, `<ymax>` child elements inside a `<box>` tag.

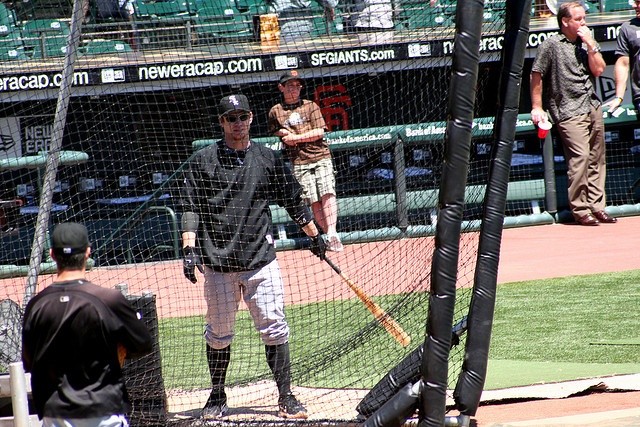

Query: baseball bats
<box><xmin>324</xmin><ymin>255</ymin><xmax>411</xmax><ymax>348</ymax></box>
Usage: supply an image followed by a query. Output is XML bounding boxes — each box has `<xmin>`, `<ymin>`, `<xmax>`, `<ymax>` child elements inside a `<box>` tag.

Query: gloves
<box><xmin>309</xmin><ymin>235</ymin><xmax>326</xmax><ymax>260</ymax></box>
<box><xmin>182</xmin><ymin>246</ymin><xmax>204</xmax><ymax>283</ymax></box>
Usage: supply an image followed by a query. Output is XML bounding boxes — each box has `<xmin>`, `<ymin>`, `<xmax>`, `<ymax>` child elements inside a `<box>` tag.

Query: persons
<box><xmin>22</xmin><ymin>222</ymin><xmax>153</xmax><ymax>427</ymax></box>
<box><xmin>531</xmin><ymin>1</ymin><xmax>618</xmax><ymax>225</ymax></box>
<box><xmin>600</xmin><ymin>0</ymin><xmax>639</xmax><ymax>114</ymax></box>
<box><xmin>181</xmin><ymin>94</ymin><xmax>326</xmax><ymax>420</ymax></box>
<box><xmin>326</xmin><ymin>0</ymin><xmax>437</xmax><ymax>34</ymax></box>
<box><xmin>91</xmin><ymin>1</ymin><xmax>139</xmax><ymax>53</ymax></box>
<box><xmin>265</xmin><ymin>0</ymin><xmax>337</xmax><ymax>42</ymax></box>
<box><xmin>267</xmin><ymin>69</ymin><xmax>343</xmax><ymax>251</ymax></box>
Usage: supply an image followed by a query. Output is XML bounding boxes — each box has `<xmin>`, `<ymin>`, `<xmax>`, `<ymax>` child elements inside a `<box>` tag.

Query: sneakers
<box><xmin>321</xmin><ymin>232</ymin><xmax>344</xmax><ymax>251</ymax></box>
<box><xmin>277</xmin><ymin>390</ymin><xmax>308</xmax><ymax>418</ymax></box>
<box><xmin>199</xmin><ymin>392</ymin><xmax>229</xmax><ymax>419</ymax></box>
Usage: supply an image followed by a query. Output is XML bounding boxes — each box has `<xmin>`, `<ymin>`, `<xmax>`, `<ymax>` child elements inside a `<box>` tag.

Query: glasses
<box><xmin>221</xmin><ymin>115</ymin><xmax>249</xmax><ymax>122</ymax></box>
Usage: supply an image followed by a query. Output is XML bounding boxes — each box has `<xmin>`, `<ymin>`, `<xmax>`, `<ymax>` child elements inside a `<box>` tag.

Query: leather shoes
<box><xmin>592</xmin><ymin>210</ymin><xmax>617</xmax><ymax>223</ymax></box>
<box><xmin>575</xmin><ymin>213</ymin><xmax>600</xmax><ymax>225</ymax></box>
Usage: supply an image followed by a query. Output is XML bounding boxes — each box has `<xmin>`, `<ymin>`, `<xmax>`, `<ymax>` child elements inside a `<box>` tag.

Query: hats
<box><xmin>220</xmin><ymin>94</ymin><xmax>251</xmax><ymax>118</ymax></box>
<box><xmin>280</xmin><ymin>70</ymin><xmax>302</xmax><ymax>83</ymax></box>
<box><xmin>51</xmin><ymin>220</ymin><xmax>93</xmax><ymax>256</ymax></box>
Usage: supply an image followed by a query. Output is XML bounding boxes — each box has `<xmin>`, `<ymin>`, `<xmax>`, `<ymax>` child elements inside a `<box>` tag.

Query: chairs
<box><xmin>0</xmin><ymin>0</ymin><xmax>135</xmax><ymax>62</ymax></box>
<box><xmin>136</xmin><ymin>2</ymin><xmax>344</xmax><ymax>38</ymax></box>
<box><xmin>393</xmin><ymin>1</ymin><xmax>536</xmax><ymax>31</ymax></box>
<box><xmin>582</xmin><ymin>1</ymin><xmax>631</xmax><ymax>13</ymax></box>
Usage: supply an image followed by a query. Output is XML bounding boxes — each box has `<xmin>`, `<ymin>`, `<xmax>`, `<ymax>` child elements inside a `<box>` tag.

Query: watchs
<box><xmin>585</xmin><ymin>44</ymin><xmax>600</xmax><ymax>54</ymax></box>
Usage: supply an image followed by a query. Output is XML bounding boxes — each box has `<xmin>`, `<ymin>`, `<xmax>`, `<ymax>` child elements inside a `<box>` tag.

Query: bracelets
<box><xmin>612</xmin><ymin>95</ymin><xmax>624</xmax><ymax>103</ymax></box>
